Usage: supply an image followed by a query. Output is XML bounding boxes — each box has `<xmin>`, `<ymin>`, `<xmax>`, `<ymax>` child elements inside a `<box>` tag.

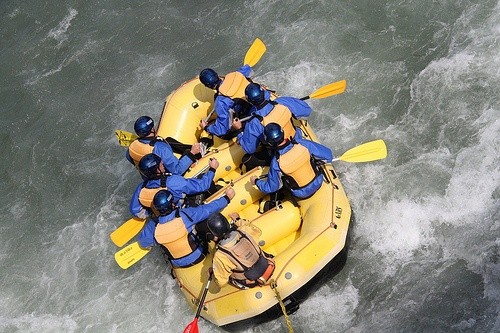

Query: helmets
<box><xmin>153</xmin><ymin>189</ymin><xmax>173</xmax><ymax>213</ymax></box>
<box><xmin>134</xmin><ymin>116</ymin><xmax>154</xmax><ymax>138</ymax></box>
<box><xmin>199</xmin><ymin>68</ymin><xmax>219</xmax><ymax>89</ymax></box>
<box><xmin>245</xmin><ymin>83</ymin><xmax>264</xmax><ymax>106</ymax></box>
<box><xmin>138</xmin><ymin>153</ymin><xmax>161</xmax><ymax>179</ymax></box>
<box><xmin>263</xmin><ymin>123</ymin><xmax>284</xmax><ymax>147</ymax></box>
<box><xmin>207</xmin><ymin>213</ymin><xmax>230</xmax><ymax>237</ymax></box>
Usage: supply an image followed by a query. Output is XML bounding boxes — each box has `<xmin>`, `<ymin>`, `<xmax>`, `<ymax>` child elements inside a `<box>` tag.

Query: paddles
<box><xmin>237</xmin><ymin>80</ymin><xmax>346</xmax><ymax>125</ymax></box>
<box><xmin>201</xmin><ymin>38</ymin><xmax>267</xmax><ymax>126</ymax></box>
<box><xmin>182</xmin><ymin>271</ymin><xmax>215</xmax><ymax>333</ymax></box>
<box><xmin>109</xmin><ymin>157</ymin><xmax>215</xmax><ymax>249</ymax></box>
<box><xmin>114</xmin><ymin>181</ymin><xmax>232</xmax><ymax>271</ymax></box>
<box><xmin>257</xmin><ymin>139</ymin><xmax>388</xmax><ymax>181</ymax></box>
<box><xmin>114</xmin><ymin>128</ymin><xmax>193</xmax><ymax>151</ymax></box>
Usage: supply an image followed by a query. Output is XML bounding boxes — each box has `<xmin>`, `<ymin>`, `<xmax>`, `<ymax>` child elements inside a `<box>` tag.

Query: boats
<box><xmin>145</xmin><ymin>72</ymin><xmax>358</xmax><ymax>333</ymax></box>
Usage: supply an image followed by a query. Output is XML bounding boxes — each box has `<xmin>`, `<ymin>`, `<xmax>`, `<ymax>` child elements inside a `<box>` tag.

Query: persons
<box><xmin>205</xmin><ymin>213</ymin><xmax>275</xmax><ymax>289</ymax></box>
<box><xmin>140</xmin><ymin>190</ymin><xmax>236</xmax><ymax>267</ymax></box>
<box><xmin>131</xmin><ymin>153</ymin><xmax>220</xmax><ymax>221</ymax></box>
<box><xmin>250</xmin><ymin>122</ymin><xmax>334</xmax><ymax>200</ymax></box>
<box><xmin>126</xmin><ymin>116</ymin><xmax>203</xmax><ymax>176</ymax></box>
<box><xmin>229</xmin><ymin>83</ymin><xmax>313</xmax><ymax>169</ymax></box>
<box><xmin>200</xmin><ymin>65</ymin><xmax>254</xmax><ymax>140</ymax></box>
